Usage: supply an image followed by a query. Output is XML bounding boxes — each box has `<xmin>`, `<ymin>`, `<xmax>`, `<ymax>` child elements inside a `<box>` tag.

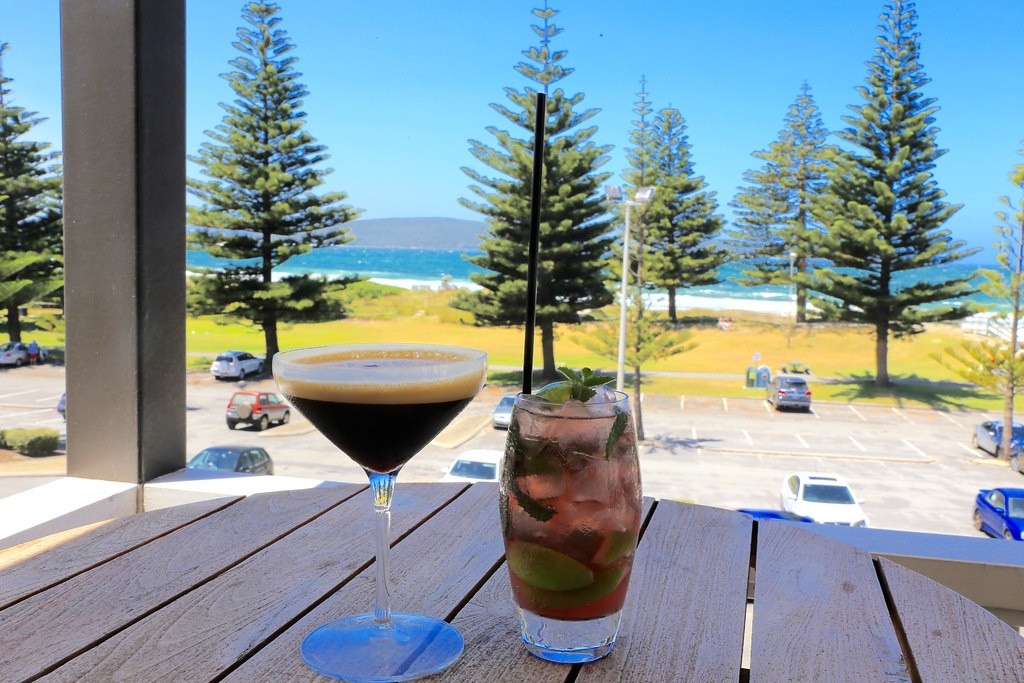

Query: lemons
<box><xmin>505</xmin><ymin>531</ymin><xmax>635</xmax><ymax>608</ymax></box>
<box><xmin>537</xmin><ymin>380</ymin><xmax>573</xmax><ymax>404</ymax></box>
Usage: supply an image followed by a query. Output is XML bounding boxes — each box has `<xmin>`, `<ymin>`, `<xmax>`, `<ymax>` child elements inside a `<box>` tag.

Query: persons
<box><xmin>27</xmin><ymin>341</ymin><xmax>40</xmax><ymax>366</ymax></box>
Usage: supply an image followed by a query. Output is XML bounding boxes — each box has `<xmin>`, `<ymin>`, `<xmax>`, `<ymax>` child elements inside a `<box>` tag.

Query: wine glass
<box><xmin>273</xmin><ymin>343</ymin><xmax>488</xmax><ymax>683</ymax></box>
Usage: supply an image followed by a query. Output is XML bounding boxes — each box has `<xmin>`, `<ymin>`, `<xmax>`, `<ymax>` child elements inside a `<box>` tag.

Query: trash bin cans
<box><xmin>746</xmin><ymin>364</ymin><xmax>772</xmax><ymax>389</ymax></box>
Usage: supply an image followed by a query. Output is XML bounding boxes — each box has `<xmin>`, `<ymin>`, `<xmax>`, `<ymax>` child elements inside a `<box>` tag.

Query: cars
<box><xmin>491</xmin><ymin>392</ymin><xmax>520</xmax><ymax>429</ymax></box>
<box><xmin>0</xmin><ymin>341</ymin><xmax>49</xmax><ymax>367</ymax></box>
<box><xmin>186</xmin><ymin>445</ymin><xmax>274</xmax><ymax>475</ymax></box>
<box><xmin>775</xmin><ymin>471</ymin><xmax>867</xmax><ymax>528</ymax></box>
<box><xmin>973</xmin><ymin>487</ymin><xmax>1024</xmax><ymax>542</ymax></box>
<box><xmin>971</xmin><ymin>420</ymin><xmax>1024</xmax><ymax>475</ymax></box>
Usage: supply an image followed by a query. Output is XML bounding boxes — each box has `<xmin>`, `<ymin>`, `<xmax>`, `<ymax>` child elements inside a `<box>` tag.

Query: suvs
<box><xmin>210</xmin><ymin>350</ymin><xmax>266</xmax><ymax>381</ymax></box>
<box><xmin>226</xmin><ymin>390</ymin><xmax>291</xmax><ymax>431</ymax></box>
<box><xmin>766</xmin><ymin>375</ymin><xmax>812</xmax><ymax>412</ymax></box>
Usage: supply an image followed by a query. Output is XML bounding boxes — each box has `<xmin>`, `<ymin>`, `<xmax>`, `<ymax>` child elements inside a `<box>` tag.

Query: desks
<box><xmin>1</xmin><ymin>483</ymin><xmax>1024</xmax><ymax>683</ymax></box>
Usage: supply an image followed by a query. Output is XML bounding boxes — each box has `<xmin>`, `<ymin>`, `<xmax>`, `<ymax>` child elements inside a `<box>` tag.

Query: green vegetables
<box><xmin>498</xmin><ymin>366</ymin><xmax>630</xmax><ymax>542</ymax></box>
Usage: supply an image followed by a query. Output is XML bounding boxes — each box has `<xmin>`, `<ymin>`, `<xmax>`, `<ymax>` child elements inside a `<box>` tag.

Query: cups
<box><xmin>498</xmin><ymin>389</ymin><xmax>641</xmax><ymax>664</ymax></box>
